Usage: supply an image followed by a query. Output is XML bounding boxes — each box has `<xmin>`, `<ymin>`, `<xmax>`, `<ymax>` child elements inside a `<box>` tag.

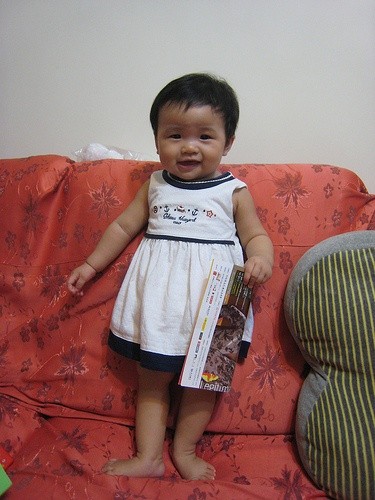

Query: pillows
<box><xmin>284</xmin><ymin>230</ymin><xmax>375</xmax><ymax>500</ymax></box>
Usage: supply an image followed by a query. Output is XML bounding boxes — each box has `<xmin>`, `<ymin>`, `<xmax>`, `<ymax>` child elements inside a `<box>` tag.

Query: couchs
<box><xmin>0</xmin><ymin>154</ymin><xmax>375</xmax><ymax>500</ymax></box>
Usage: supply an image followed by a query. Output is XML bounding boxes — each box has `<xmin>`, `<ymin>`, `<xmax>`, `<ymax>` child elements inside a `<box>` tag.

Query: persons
<box><xmin>67</xmin><ymin>74</ymin><xmax>275</xmax><ymax>482</ymax></box>
